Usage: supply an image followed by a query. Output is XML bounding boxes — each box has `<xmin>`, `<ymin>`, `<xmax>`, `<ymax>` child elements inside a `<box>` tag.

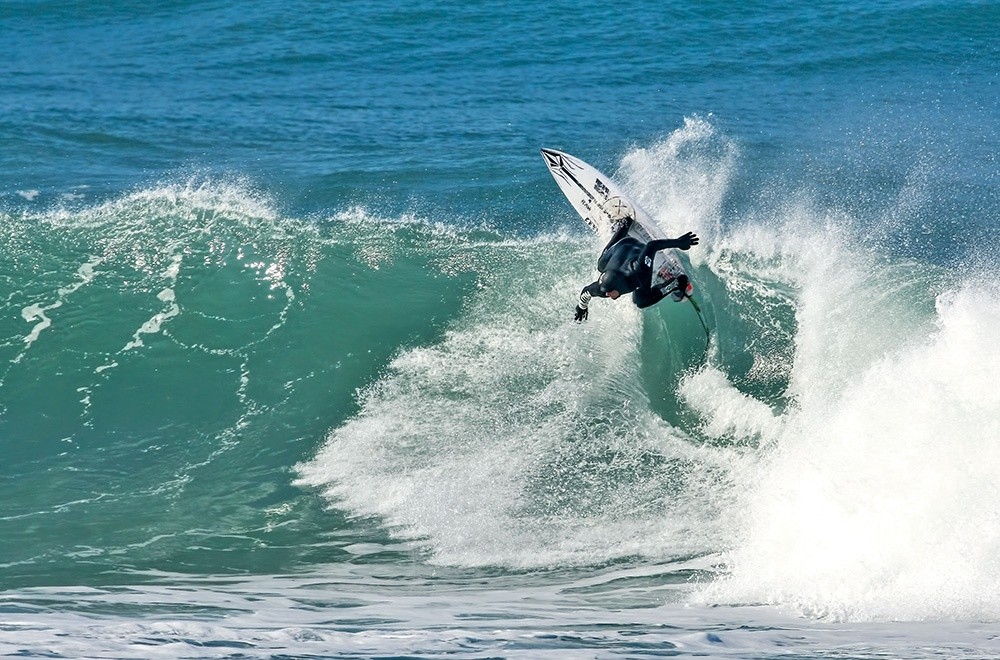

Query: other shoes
<box><xmin>620</xmin><ymin>217</ymin><xmax>633</xmax><ymax>237</ymax></box>
<box><xmin>672</xmin><ymin>275</ymin><xmax>688</xmax><ymax>302</ymax></box>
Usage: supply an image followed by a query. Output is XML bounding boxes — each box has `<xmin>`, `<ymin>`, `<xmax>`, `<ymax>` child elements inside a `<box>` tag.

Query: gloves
<box><xmin>678</xmin><ymin>232</ymin><xmax>699</xmax><ymax>250</ymax></box>
<box><xmin>575</xmin><ymin>306</ymin><xmax>587</xmax><ymax>322</ymax></box>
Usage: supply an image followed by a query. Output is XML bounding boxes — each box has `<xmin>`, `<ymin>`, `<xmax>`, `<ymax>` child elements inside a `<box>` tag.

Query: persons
<box><xmin>574</xmin><ymin>213</ymin><xmax>699</xmax><ymax>322</ymax></box>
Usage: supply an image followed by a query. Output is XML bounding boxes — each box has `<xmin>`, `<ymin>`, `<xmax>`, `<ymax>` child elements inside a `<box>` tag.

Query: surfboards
<box><xmin>537</xmin><ymin>148</ymin><xmax>694</xmax><ymax>304</ymax></box>
